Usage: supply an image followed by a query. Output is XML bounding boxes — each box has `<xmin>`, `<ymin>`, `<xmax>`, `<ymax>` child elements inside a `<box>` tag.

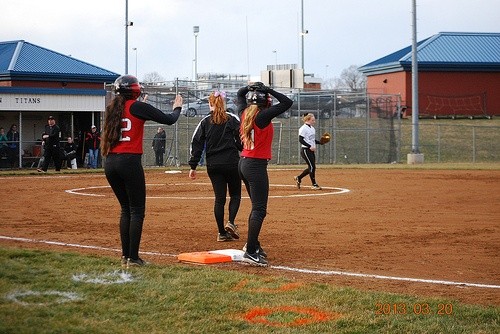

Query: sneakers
<box><xmin>243</xmin><ymin>253</ymin><xmax>267</xmax><ymax>266</ymax></box>
<box><xmin>120</xmin><ymin>255</ymin><xmax>127</xmax><ymax>263</ymax></box>
<box><xmin>294</xmin><ymin>176</ymin><xmax>301</xmax><ymax>189</ymax></box>
<box><xmin>312</xmin><ymin>185</ymin><xmax>321</xmax><ymax>189</ymax></box>
<box><xmin>218</xmin><ymin>232</ymin><xmax>233</xmax><ymax>241</ymax></box>
<box><xmin>127</xmin><ymin>257</ymin><xmax>148</xmax><ymax>266</ymax></box>
<box><xmin>242</xmin><ymin>242</ymin><xmax>267</xmax><ymax>258</ymax></box>
<box><xmin>225</xmin><ymin>221</ymin><xmax>240</xmax><ymax>239</ymax></box>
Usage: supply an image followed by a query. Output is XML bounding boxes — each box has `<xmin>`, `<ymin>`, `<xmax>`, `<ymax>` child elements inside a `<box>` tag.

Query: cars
<box><xmin>179</xmin><ymin>96</ymin><xmax>238</xmax><ymax>117</ymax></box>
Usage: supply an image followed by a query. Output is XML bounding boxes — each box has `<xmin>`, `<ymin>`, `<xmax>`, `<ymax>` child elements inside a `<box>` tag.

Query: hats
<box><xmin>48</xmin><ymin>116</ymin><xmax>55</xmax><ymax>120</ymax></box>
<box><xmin>92</xmin><ymin>126</ymin><xmax>95</xmax><ymax>128</ymax></box>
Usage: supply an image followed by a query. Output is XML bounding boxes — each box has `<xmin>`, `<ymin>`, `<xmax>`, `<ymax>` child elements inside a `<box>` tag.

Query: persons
<box><xmin>152</xmin><ymin>127</ymin><xmax>166</xmax><ymax>165</ymax></box>
<box><xmin>7</xmin><ymin>124</ymin><xmax>19</xmax><ymax>168</ymax></box>
<box><xmin>188</xmin><ymin>90</ymin><xmax>244</xmax><ymax>242</ymax></box>
<box><xmin>103</xmin><ymin>75</ymin><xmax>183</xmax><ymax>268</ymax></box>
<box><xmin>85</xmin><ymin>125</ymin><xmax>101</xmax><ymax>169</ymax></box>
<box><xmin>235</xmin><ymin>82</ymin><xmax>294</xmax><ymax>266</ymax></box>
<box><xmin>294</xmin><ymin>113</ymin><xmax>322</xmax><ymax>190</ymax></box>
<box><xmin>0</xmin><ymin>128</ymin><xmax>8</xmax><ymax>168</ymax></box>
<box><xmin>43</xmin><ymin>116</ymin><xmax>61</xmax><ymax>172</ymax></box>
<box><xmin>61</xmin><ymin>136</ymin><xmax>79</xmax><ymax>169</ymax></box>
<box><xmin>30</xmin><ymin>132</ymin><xmax>48</xmax><ymax>172</ymax></box>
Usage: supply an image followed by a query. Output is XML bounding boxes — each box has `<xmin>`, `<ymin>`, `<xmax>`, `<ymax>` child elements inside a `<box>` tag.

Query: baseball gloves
<box><xmin>320</xmin><ymin>133</ymin><xmax>330</xmax><ymax>144</ymax></box>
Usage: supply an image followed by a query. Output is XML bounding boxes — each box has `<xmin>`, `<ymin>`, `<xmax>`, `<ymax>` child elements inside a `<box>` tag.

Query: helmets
<box><xmin>246</xmin><ymin>88</ymin><xmax>273</xmax><ymax>108</ymax></box>
<box><xmin>113</xmin><ymin>75</ymin><xmax>149</xmax><ymax>102</ymax></box>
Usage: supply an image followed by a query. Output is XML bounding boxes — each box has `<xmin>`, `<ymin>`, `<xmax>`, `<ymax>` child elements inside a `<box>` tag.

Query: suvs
<box><xmin>272</xmin><ymin>91</ymin><xmax>341</xmax><ymax>119</ymax></box>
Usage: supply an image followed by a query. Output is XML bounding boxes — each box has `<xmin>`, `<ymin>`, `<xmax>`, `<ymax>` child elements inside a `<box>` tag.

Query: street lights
<box><xmin>132</xmin><ymin>47</ymin><xmax>137</xmax><ymax>77</ymax></box>
<box><xmin>300</xmin><ymin>0</ymin><xmax>309</xmax><ymax>70</ymax></box>
<box><xmin>273</xmin><ymin>50</ymin><xmax>277</xmax><ymax>71</ymax></box>
<box><xmin>193</xmin><ymin>26</ymin><xmax>199</xmax><ymax>101</ymax></box>
<box><xmin>124</xmin><ymin>0</ymin><xmax>133</xmax><ymax>75</ymax></box>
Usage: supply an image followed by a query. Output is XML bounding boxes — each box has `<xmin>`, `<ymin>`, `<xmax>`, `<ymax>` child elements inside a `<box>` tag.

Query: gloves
<box><xmin>247</xmin><ymin>82</ymin><xmax>267</xmax><ymax>92</ymax></box>
